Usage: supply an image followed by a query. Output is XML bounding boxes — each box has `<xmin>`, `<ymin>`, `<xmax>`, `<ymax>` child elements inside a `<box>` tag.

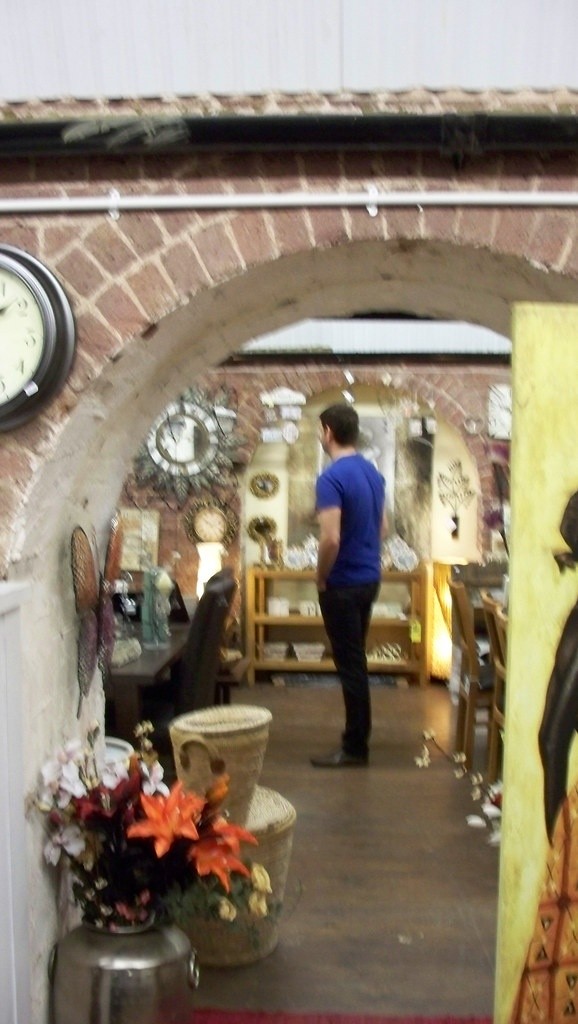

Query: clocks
<box><xmin>185</xmin><ymin>498</ymin><xmax>238</xmax><ymax>547</ymax></box>
<box><xmin>0</xmin><ymin>242</ymin><xmax>75</xmax><ymax>433</ymax></box>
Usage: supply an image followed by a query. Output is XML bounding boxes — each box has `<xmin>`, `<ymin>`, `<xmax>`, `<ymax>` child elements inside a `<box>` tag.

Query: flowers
<box><xmin>25</xmin><ymin>719</ymin><xmax>281</xmax><ymax>954</ymax></box>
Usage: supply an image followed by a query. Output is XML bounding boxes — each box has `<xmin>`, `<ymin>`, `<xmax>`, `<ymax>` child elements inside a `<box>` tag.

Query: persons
<box><xmin>308</xmin><ymin>403</ymin><xmax>387</xmax><ymax>769</ymax></box>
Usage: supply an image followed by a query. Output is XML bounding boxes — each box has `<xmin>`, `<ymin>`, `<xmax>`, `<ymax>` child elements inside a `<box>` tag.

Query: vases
<box><xmin>49</xmin><ymin>912</ymin><xmax>201</xmax><ymax>1024</ymax></box>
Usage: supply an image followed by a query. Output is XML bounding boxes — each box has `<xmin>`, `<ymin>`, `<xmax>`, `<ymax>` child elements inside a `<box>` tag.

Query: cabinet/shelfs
<box><xmin>245</xmin><ymin>564</ymin><xmax>433</xmax><ymax>688</ymax></box>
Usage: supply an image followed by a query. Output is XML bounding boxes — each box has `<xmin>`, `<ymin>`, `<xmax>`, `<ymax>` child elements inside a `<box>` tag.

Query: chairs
<box><xmin>480</xmin><ymin>588</ymin><xmax>508</xmax><ymax>782</ymax></box>
<box><xmin>146</xmin><ymin>568</ymin><xmax>239</xmax><ymax>738</ymax></box>
<box><xmin>447</xmin><ymin>577</ymin><xmax>493</xmax><ymax>768</ymax></box>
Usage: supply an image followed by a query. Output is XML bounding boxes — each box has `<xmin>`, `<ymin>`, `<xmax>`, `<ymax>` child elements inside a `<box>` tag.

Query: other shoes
<box><xmin>310</xmin><ymin>747</ymin><xmax>370</xmax><ymax>770</ymax></box>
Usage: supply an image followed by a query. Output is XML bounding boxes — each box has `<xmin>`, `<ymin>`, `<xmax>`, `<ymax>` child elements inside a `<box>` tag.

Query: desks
<box><xmin>107</xmin><ymin>623</ymin><xmax>192</xmax><ymax>749</ymax></box>
